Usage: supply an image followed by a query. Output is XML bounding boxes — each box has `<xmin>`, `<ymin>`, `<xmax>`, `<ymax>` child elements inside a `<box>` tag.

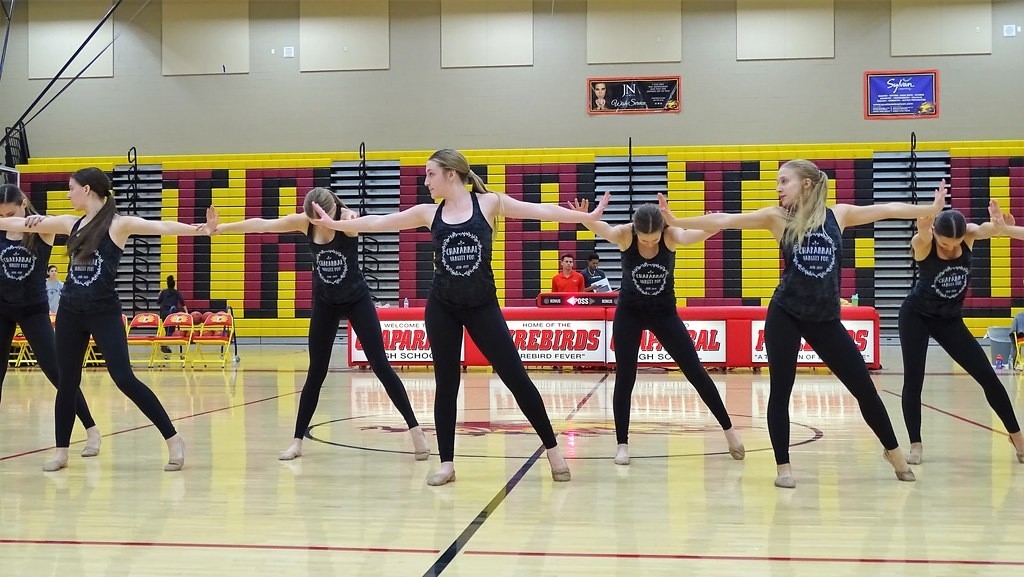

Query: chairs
<box><xmin>6</xmin><ymin>311</ymin><xmax>236</xmax><ymax>370</ymax></box>
<box><xmin>1012</xmin><ymin>313</ymin><xmax>1024</xmax><ymax>371</ymax></box>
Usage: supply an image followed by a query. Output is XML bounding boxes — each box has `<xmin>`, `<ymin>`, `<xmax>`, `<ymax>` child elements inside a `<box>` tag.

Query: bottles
<box><xmin>1008</xmin><ymin>355</ymin><xmax>1014</xmax><ymax>370</ymax></box>
<box><xmin>231</xmin><ymin>354</ymin><xmax>236</xmax><ymax>367</ymax></box>
<box><xmin>996</xmin><ymin>354</ymin><xmax>1003</xmax><ymax>369</ymax></box>
<box><xmin>404</xmin><ymin>298</ymin><xmax>409</xmax><ymax>308</ymax></box>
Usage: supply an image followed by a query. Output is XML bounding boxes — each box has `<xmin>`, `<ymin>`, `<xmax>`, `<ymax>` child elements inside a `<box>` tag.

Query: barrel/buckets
<box><xmin>987</xmin><ymin>326</ymin><xmax>1016</xmax><ymax>365</ymax></box>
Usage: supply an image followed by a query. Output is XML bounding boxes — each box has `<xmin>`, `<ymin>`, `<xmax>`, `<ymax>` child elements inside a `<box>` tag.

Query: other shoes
<box><xmin>730</xmin><ymin>444</ymin><xmax>744</xmax><ymax>460</ymax></box>
<box><xmin>907</xmin><ymin>447</ymin><xmax>923</xmax><ymax>464</ymax></box>
<box><xmin>43</xmin><ymin>457</ymin><xmax>69</xmax><ymax>471</ymax></box>
<box><xmin>547</xmin><ymin>453</ymin><xmax>571</xmax><ymax>482</ymax></box>
<box><xmin>615</xmin><ymin>456</ymin><xmax>630</xmax><ymax>465</ymax></box>
<box><xmin>81</xmin><ymin>435</ymin><xmax>101</xmax><ymax>456</ymax></box>
<box><xmin>415</xmin><ymin>433</ymin><xmax>429</xmax><ymax>462</ymax></box>
<box><xmin>427</xmin><ymin>471</ymin><xmax>455</xmax><ymax>485</ymax></box>
<box><xmin>1009</xmin><ymin>435</ymin><xmax>1024</xmax><ymax>463</ymax></box>
<box><xmin>776</xmin><ymin>477</ymin><xmax>795</xmax><ymax>488</ymax></box>
<box><xmin>279</xmin><ymin>449</ymin><xmax>301</xmax><ymax>459</ymax></box>
<box><xmin>164</xmin><ymin>440</ymin><xmax>185</xmax><ymax>471</ymax></box>
<box><xmin>883</xmin><ymin>449</ymin><xmax>915</xmax><ymax>481</ymax></box>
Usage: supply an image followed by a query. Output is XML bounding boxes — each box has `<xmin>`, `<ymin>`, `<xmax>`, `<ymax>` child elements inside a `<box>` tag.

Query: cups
<box><xmin>851</xmin><ymin>295</ymin><xmax>859</xmax><ymax>306</ymax></box>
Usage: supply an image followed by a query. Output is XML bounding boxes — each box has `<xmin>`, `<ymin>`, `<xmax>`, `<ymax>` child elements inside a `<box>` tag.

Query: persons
<box><xmin>592</xmin><ymin>82</ymin><xmax>611</xmax><ymax>109</ymax></box>
<box><xmin>898</xmin><ymin>211</ymin><xmax>1024</xmax><ymax>464</ymax></box>
<box><xmin>192</xmin><ymin>186</ymin><xmax>431</xmax><ymax>462</ymax></box>
<box><xmin>0</xmin><ymin>184</ymin><xmax>101</xmax><ymax>456</ymax></box>
<box><xmin>157</xmin><ymin>275</ymin><xmax>188</xmax><ymax>354</ymax></box>
<box><xmin>551</xmin><ymin>254</ymin><xmax>585</xmax><ymax>293</ymax></box>
<box><xmin>580</xmin><ymin>253</ymin><xmax>613</xmax><ymax>292</ymax></box>
<box><xmin>45</xmin><ymin>265</ymin><xmax>64</xmax><ymax>314</ymax></box>
<box><xmin>657</xmin><ymin>159</ymin><xmax>947</xmax><ymax>488</ymax></box>
<box><xmin>310</xmin><ymin>148</ymin><xmax>611</xmax><ymax>486</ymax></box>
<box><xmin>0</xmin><ymin>167</ymin><xmax>220</xmax><ymax>472</ymax></box>
<box><xmin>568</xmin><ymin>198</ymin><xmax>745</xmax><ymax>464</ymax></box>
<box><xmin>988</xmin><ymin>199</ymin><xmax>1024</xmax><ymax>241</ymax></box>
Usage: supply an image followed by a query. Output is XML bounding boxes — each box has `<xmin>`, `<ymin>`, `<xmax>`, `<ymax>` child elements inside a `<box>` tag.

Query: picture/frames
<box><xmin>863</xmin><ymin>69</ymin><xmax>939</xmax><ymax>121</ymax></box>
<box><xmin>587</xmin><ymin>76</ymin><xmax>681</xmax><ymax>115</ymax></box>
